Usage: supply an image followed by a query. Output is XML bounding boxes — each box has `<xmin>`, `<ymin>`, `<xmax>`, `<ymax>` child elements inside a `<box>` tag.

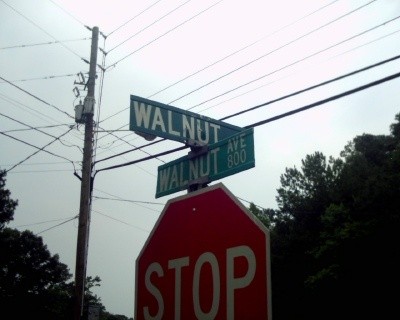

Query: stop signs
<box><xmin>134</xmin><ymin>183</ymin><xmax>273</xmax><ymax>320</ymax></box>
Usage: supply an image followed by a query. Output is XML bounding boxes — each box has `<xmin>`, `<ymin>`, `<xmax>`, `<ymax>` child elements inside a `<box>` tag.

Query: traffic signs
<box><xmin>155</xmin><ymin>127</ymin><xmax>255</xmax><ymax>199</ymax></box>
<box><xmin>130</xmin><ymin>94</ymin><xmax>246</xmax><ymax>147</ymax></box>
